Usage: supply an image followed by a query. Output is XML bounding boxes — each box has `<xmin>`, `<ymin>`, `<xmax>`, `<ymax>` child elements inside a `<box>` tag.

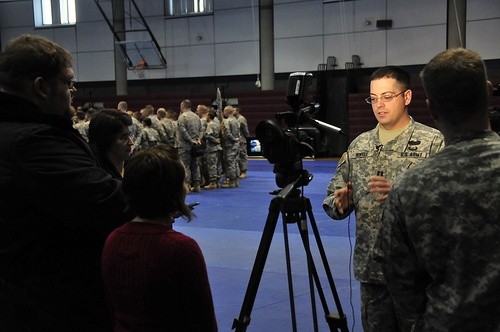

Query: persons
<box><xmin>69</xmin><ymin>101</ymin><xmax>249</xmax><ymax>189</ymax></box>
<box><xmin>323</xmin><ymin>67</ymin><xmax>447</xmax><ymax>332</ymax></box>
<box><xmin>380</xmin><ymin>48</ymin><xmax>500</xmax><ymax>332</ymax></box>
<box><xmin>101</xmin><ymin>149</ymin><xmax>219</xmax><ymax>332</ymax></box>
<box><xmin>87</xmin><ymin>109</ymin><xmax>134</xmax><ymax>177</ymax></box>
<box><xmin>0</xmin><ymin>35</ymin><xmax>197</xmax><ymax>332</ymax></box>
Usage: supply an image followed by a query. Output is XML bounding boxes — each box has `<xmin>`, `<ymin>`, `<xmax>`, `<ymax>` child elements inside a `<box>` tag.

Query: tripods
<box><xmin>232</xmin><ymin>189</ymin><xmax>351</xmax><ymax>332</ymax></box>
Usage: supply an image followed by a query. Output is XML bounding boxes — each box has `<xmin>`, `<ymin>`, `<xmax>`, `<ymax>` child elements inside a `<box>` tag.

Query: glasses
<box><xmin>366</xmin><ymin>90</ymin><xmax>406</xmax><ymax>104</ymax></box>
<box><xmin>116</xmin><ymin>132</ymin><xmax>136</xmax><ymax>143</ymax></box>
<box><xmin>55</xmin><ymin>74</ymin><xmax>78</xmax><ymax>88</ymax></box>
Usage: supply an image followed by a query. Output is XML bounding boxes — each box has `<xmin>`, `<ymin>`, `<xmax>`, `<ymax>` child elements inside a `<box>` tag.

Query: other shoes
<box><xmin>216</xmin><ymin>179</ymin><xmax>221</xmax><ymax>187</ymax></box>
<box><xmin>185</xmin><ymin>182</ymin><xmax>191</xmax><ymax>193</ymax></box>
<box><xmin>201</xmin><ymin>174</ymin><xmax>210</xmax><ymax>187</ymax></box>
<box><xmin>190</xmin><ymin>181</ymin><xmax>201</xmax><ymax>192</ymax></box>
<box><xmin>204</xmin><ymin>181</ymin><xmax>216</xmax><ymax>189</ymax></box>
<box><xmin>240</xmin><ymin>171</ymin><xmax>247</xmax><ymax>178</ymax></box>
<box><xmin>221</xmin><ymin>179</ymin><xmax>238</xmax><ymax>188</ymax></box>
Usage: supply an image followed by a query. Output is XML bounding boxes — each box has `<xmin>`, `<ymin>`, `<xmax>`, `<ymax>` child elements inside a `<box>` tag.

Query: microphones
<box><xmin>376</xmin><ymin>145</ymin><xmax>383</xmax><ymax>148</ymax></box>
<box><xmin>298</xmin><ymin>102</ymin><xmax>320</xmax><ymax>116</ymax></box>
